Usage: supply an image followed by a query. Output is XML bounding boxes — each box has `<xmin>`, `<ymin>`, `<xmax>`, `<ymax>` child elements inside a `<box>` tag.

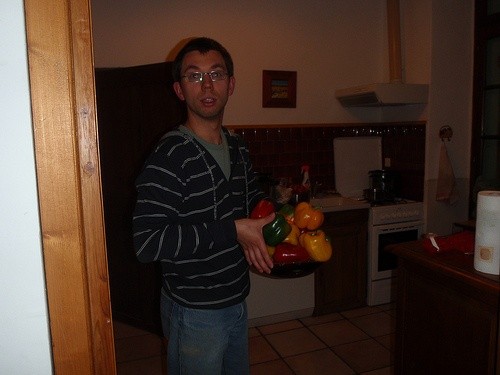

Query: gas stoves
<box><xmin>354</xmin><ymin>197</ymin><xmax>423</xmax><ymax>225</ymax></box>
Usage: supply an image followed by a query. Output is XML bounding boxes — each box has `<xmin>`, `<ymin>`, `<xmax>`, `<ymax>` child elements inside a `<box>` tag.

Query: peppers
<box><xmin>252</xmin><ymin>200</ymin><xmax>332</xmax><ymax>264</ymax></box>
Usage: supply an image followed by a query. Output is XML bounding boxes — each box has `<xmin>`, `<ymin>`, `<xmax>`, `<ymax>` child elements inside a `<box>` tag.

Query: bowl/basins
<box><xmin>252</xmin><ymin>261</ymin><xmax>320</xmax><ymax>277</ymax></box>
<box><xmin>292</xmin><ymin>193</ymin><xmax>308</xmax><ymax>204</ymax></box>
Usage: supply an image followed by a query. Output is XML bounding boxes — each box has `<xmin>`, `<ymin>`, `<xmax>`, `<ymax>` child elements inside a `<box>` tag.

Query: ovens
<box><xmin>367</xmin><ymin>220</ymin><xmax>423</xmax><ymax>306</ymax></box>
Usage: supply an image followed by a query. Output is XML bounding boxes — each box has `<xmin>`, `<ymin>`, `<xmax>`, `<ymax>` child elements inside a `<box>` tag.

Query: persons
<box><xmin>133</xmin><ymin>38</ymin><xmax>276</xmax><ymax>375</ymax></box>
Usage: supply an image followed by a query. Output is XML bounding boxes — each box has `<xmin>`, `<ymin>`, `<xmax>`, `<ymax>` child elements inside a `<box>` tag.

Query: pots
<box><xmin>370</xmin><ymin>170</ymin><xmax>395</xmax><ymax>200</ymax></box>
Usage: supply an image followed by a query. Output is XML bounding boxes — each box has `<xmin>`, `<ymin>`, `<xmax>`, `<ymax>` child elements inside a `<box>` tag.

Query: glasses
<box><xmin>179</xmin><ymin>71</ymin><xmax>229</xmax><ymax>83</ymax></box>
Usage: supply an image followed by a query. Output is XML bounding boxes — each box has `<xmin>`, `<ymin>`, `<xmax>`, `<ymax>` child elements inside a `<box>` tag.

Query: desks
<box><xmin>386</xmin><ymin>232</ymin><xmax>500</xmax><ymax>375</ymax></box>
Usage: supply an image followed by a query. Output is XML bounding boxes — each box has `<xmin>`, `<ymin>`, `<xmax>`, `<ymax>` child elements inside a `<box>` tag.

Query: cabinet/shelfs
<box><xmin>314</xmin><ymin>208</ymin><xmax>369</xmax><ymax>316</ymax></box>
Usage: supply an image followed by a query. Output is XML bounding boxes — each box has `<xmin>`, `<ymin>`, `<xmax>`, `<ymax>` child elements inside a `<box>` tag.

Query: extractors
<box><xmin>333</xmin><ymin>0</ymin><xmax>428</xmax><ymax>103</ymax></box>
